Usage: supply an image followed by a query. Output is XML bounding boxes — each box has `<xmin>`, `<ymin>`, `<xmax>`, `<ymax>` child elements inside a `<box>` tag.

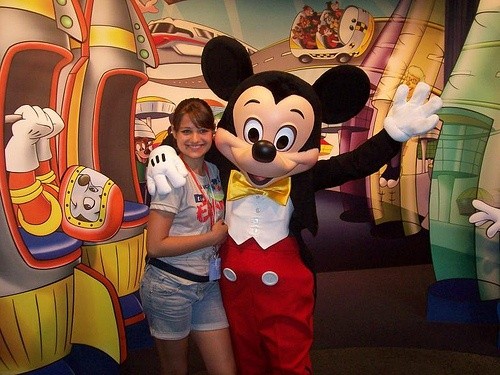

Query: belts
<box><xmin>146</xmin><ymin>254</ymin><xmax>220</xmax><ymax>283</ymax></box>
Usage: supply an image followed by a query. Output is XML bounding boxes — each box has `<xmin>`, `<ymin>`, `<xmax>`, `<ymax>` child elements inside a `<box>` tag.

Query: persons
<box><xmin>139</xmin><ymin>98</ymin><xmax>238</xmax><ymax>375</ymax></box>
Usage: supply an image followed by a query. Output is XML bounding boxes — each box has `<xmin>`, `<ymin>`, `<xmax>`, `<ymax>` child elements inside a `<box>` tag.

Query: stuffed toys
<box><xmin>146</xmin><ymin>35</ymin><xmax>442</xmax><ymax>375</ymax></box>
